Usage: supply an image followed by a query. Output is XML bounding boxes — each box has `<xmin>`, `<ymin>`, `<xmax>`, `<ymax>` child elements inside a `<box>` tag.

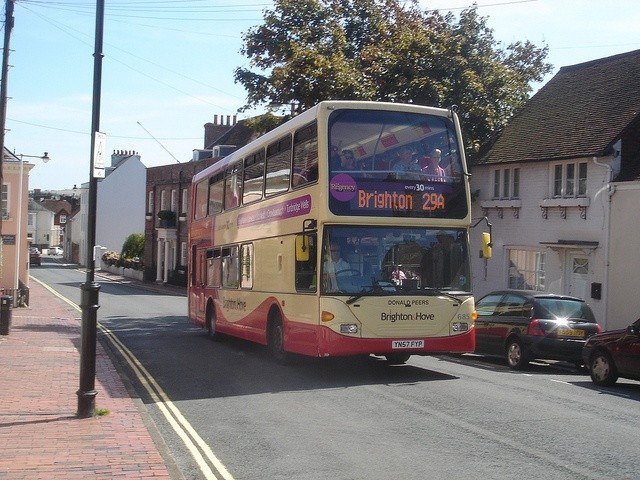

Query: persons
<box><xmin>333</xmin><ymin>150</ymin><xmax>364</xmax><ymax>178</ymax></box>
<box><xmin>310</xmin><ymin>243</ymin><xmax>352</xmax><ymax>293</ymax></box>
<box><xmin>388</xmin><ymin>145</ymin><xmax>423</xmax><ymax>181</ymax></box>
<box><xmin>420</xmin><ymin>149</ymin><xmax>448</xmax><ymax>181</ymax></box>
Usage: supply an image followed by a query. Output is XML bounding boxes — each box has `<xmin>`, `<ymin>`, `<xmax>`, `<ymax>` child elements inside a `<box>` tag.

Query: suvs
<box><xmin>475</xmin><ymin>291</ymin><xmax>602</xmax><ymax>370</ymax></box>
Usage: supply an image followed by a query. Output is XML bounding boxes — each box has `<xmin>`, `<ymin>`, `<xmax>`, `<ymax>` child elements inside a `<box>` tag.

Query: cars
<box><xmin>582</xmin><ymin>318</ymin><xmax>640</xmax><ymax>387</ymax></box>
<box><xmin>47</xmin><ymin>247</ymin><xmax>64</xmax><ymax>255</ymax></box>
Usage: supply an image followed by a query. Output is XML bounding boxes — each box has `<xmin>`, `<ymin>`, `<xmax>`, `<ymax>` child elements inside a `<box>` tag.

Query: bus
<box><xmin>184</xmin><ymin>100</ymin><xmax>492</xmax><ymax>363</ymax></box>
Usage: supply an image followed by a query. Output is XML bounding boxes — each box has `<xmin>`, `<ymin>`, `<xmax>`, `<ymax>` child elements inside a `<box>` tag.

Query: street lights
<box><xmin>14</xmin><ymin>151</ymin><xmax>51</xmax><ymax>308</ymax></box>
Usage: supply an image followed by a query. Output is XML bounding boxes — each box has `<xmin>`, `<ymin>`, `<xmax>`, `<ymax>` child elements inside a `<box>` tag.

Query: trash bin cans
<box><xmin>1</xmin><ymin>295</ymin><xmax>13</xmax><ymax>335</ymax></box>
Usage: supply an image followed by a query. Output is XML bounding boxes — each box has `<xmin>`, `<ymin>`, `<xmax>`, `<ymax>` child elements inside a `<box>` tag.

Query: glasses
<box><xmin>345</xmin><ymin>155</ymin><xmax>354</xmax><ymax>159</ymax></box>
<box><xmin>330</xmin><ymin>249</ymin><xmax>340</xmax><ymax>252</ymax></box>
